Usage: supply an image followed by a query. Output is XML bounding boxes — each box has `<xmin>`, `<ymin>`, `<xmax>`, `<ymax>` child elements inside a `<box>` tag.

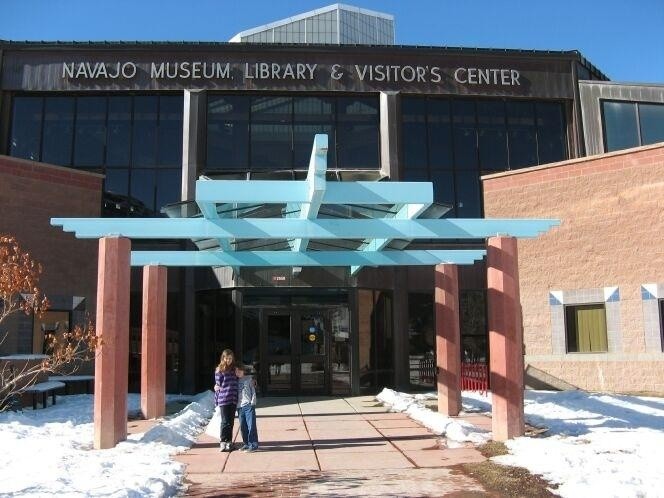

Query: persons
<box><xmin>213</xmin><ymin>365</ymin><xmax>259</xmax><ymax>453</ymax></box>
<box><xmin>214</xmin><ymin>349</ymin><xmax>257</xmax><ymax>451</ymax></box>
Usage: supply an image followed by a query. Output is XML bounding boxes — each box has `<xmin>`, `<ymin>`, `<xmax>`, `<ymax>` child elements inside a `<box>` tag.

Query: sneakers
<box><xmin>219</xmin><ymin>442</ymin><xmax>232</xmax><ymax>452</ymax></box>
<box><xmin>239</xmin><ymin>444</ymin><xmax>257</xmax><ymax>452</ymax></box>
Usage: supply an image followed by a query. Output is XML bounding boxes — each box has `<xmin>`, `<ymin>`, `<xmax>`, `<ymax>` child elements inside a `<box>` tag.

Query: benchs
<box><xmin>49</xmin><ymin>374</ymin><xmax>94</xmax><ymax>394</ymax></box>
<box><xmin>24</xmin><ymin>381</ymin><xmax>65</xmax><ymax>408</ymax></box>
